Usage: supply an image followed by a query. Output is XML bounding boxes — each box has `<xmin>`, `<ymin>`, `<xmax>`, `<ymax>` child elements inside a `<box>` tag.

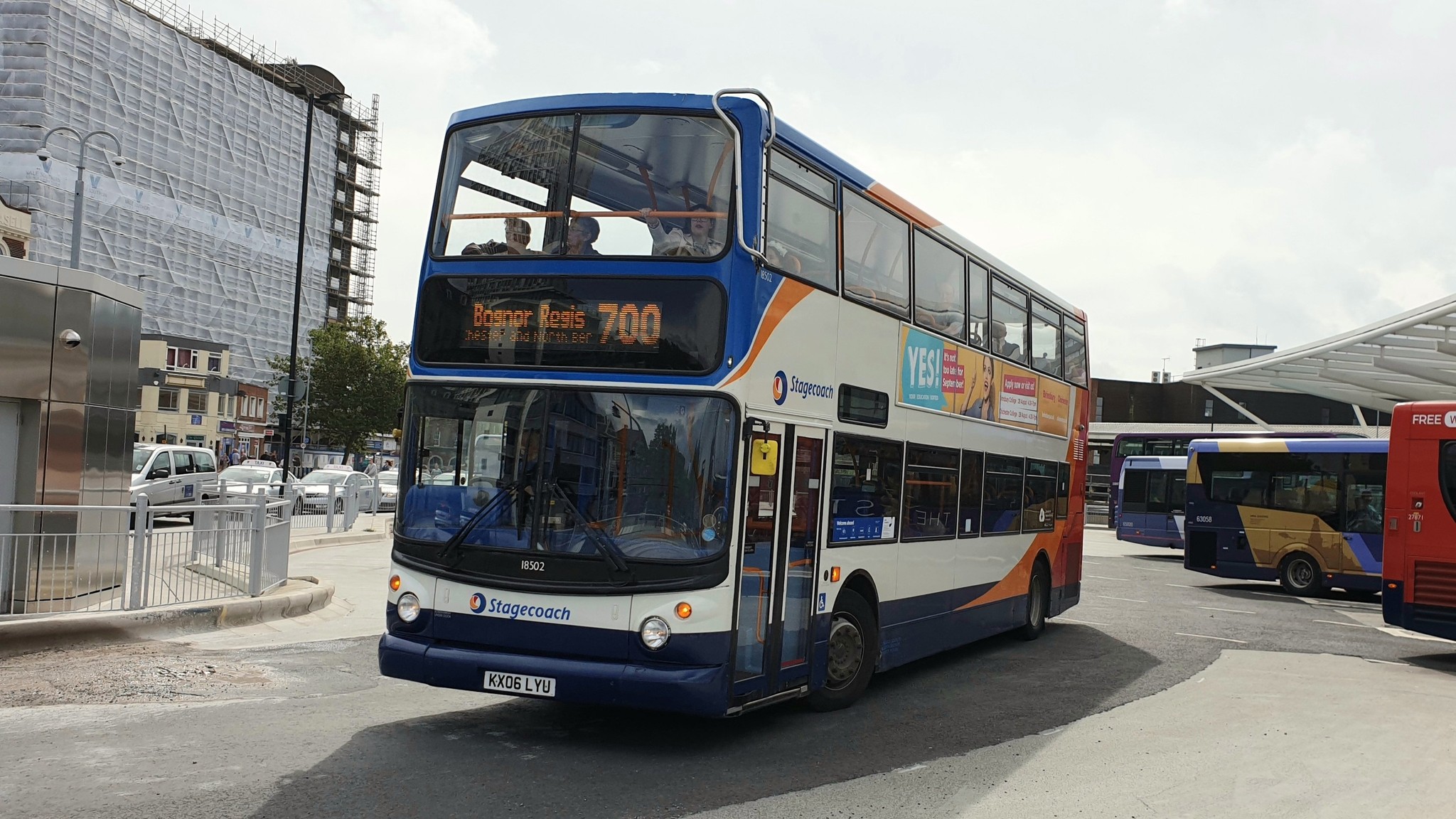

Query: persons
<box><xmin>766</xmin><ymin>240</ymin><xmax>788</xmax><ymax>270</ymax></box>
<box><xmin>461</xmin><ymin>217</ymin><xmax>602</xmax><ymax>256</ymax></box>
<box><xmin>960</xmin><ymin>356</ymin><xmax>995</xmax><ymax>423</ymax></box>
<box><xmin>841</xmin><ymin>462</ymin><xmax>1068</xmax><ymax>530</ymax></box>
<box><xmin>991</xmin><ymin>322</ymin><xmax>1022</xmax><ymax>361</ymax></box>
<box><xmin>482</xmin><ymin>430</ymin><xmax>551</xmax><ymax>527</ymax></box>
<box><xmin>382</xmin><ymin>460</ymin><xmax>392</xmax><ymax>471</ymax></box>
<box><xmin>923</xmin><ymin>281</ymin><xmax>964</xmax><ymax>338</ymax></box>
<box><xmin>639</xmin><ymin>202</ymin><xmax>725</xmax><ymax>258</ymax></box>
<box><xmin>430</xmin><ymin>463</ymin><xmax>442</xmax><ymax>477</ymax></box>
<box><xmin>292</xmin><ymin>454</ymin><xmax>301</xmax><ymax>479</ymax></box>
<box><xmin>219</xmin><ymin>448</ymin><xmax>285</xmax><ymax>471</ymax></box>
<box><xmin>358</xmin><ymin>457</ymin><xmax>377</xmax><ymax>478</ymax></box>
<box><xmin>1211</xmin><ymin>470</ymin><xmax>1382</xmax><ymax>524</ymax></box>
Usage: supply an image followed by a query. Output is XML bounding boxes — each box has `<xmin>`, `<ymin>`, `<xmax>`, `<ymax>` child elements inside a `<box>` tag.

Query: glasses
<box><xmin>691</xmin><ymin>217</ymin><xmax>709</xmax><ymax>222</ymax></box>
<box><xmin>568</xmin><ymin>226</ymin><xmax>589</xmax><ymax>233</ymax></box>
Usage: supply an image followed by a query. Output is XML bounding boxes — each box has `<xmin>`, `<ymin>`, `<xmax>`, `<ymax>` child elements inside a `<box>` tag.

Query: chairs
<box><xmin>781</xmin><ymin>252</ymin><xmax>1087</xmax><ymax>386</ymax></box>
<box><xmin>1247</xmin><ymin>486</ymin><xmax>1335</xmax><ymax>514</ymax></box>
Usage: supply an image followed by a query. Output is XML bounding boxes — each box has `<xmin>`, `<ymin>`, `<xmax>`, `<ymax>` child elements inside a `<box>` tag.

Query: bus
<box><xmin>377</xmin><ymin>89</ymin><xmax>1089</xmax><ymax>717</ymax></box>
<box><xmin>1183</xmin><ymin>438</ymin><xmax>1390</xmax><ymax>596</ymax></box>
<box><xmin>1380</xmin><ymin>401</ymin><xmax>1456</xmax><ymax>643</ymax></box>
<box><xmin>1108</xmin><ymin>432</ymin><xmax>1372</xmax><ymax>529</ymax></box>
<box><xmin>1114</xmin><ymin>455</ymin><xmax>1310</xmax><ymax>550</ymax></box>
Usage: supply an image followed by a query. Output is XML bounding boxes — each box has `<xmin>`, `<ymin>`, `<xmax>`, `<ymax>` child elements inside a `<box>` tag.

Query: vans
<box><xmin>126</xmin><ymin>442</ymin><xmax>218</xmax><ymax>530</ymax></box>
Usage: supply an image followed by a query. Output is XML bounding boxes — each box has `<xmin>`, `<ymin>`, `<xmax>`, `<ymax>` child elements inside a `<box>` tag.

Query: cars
<box><xmin>433</xmin><ymin>473</ymin><xmax>467</xmax><ymax>486</ymax></box>
<box><xmin>298</xmin><ymin>469</ymin><xmax>382</xmax><ymax>514</ymax></box>
<box><xmin>215</xmin><ymin>465</ymin><xmax>306</xmax><ymax>520</ymax></box>
<box><xmin>369</xmin><ymin>471</ymin><xmax>432</xmax><ymax>511</ymax></box>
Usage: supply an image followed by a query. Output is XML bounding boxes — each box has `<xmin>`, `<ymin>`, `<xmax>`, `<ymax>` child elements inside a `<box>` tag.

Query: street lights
<box><xmin>137</xmin><ymin>274</ymin><xmax>154</xmax><ymax>290</ymax></box>
<box><xmin>36</xmin><ymin>127</ymin><xmax>127</xmax><ymax>269</ymax></box>
<box><xmin>277</xmin><ymin>82</ymin><xmax>352</xmax><ymax>520</ymax></box>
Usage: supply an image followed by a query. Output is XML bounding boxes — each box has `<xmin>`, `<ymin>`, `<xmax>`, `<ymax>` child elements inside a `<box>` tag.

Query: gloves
<box><xmin>639</xmin><ymin>208</ymin><xmax>658</xmax><ymax>224</ymax></box>
